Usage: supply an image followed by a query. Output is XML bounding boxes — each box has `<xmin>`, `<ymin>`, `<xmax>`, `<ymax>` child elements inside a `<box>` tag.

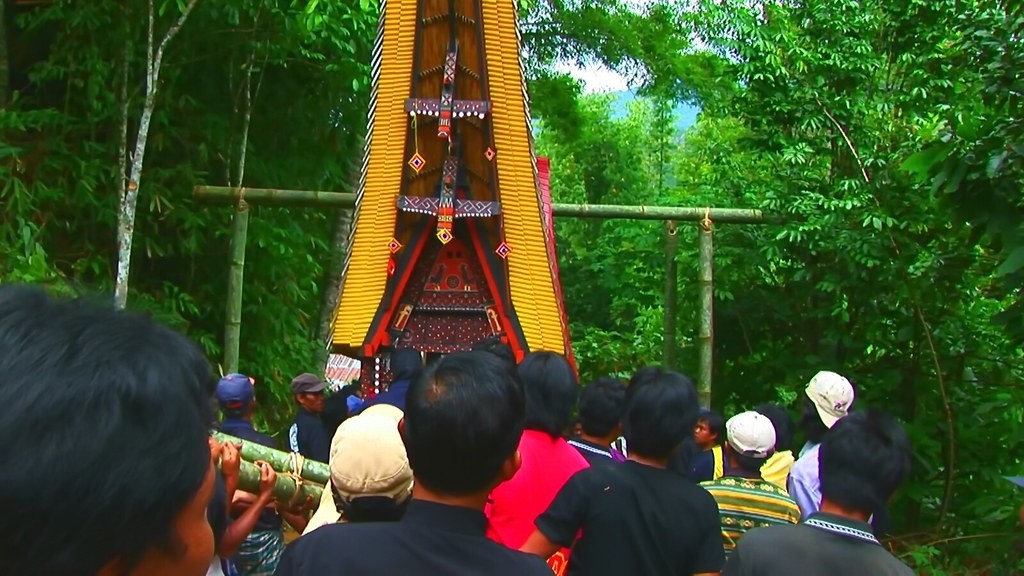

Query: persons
<box><xmin>211</xmin><ymin>338</ymin><xmax>557</xmax><ymax>576</ymax></box>
<box><xmin>690</xmin><ymin>410</ymin><xmax>917</xmax><ymax>576</ymax></box>
<box><xmin>786</xmin><ymin>370</ymin><xmax>877</xmax><ymax>525</ymax></box>
<box><xmin>483</xmin><ymin>350</ymin><xmax>726</xmax><ymax>576</ymax></box>
<box><xmin>0</xmin><ymin>280</ymin><xmax>217</xmax><ymax>576</ymax></box>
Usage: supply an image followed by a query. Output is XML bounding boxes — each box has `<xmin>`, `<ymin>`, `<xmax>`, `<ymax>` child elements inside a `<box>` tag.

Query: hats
<box><xmin>725</xmin><ymin>410</ymin><xmax>777</xmax><ymax>458</ymax></box>
<box><xmin>805</xmin><ymin>370</ymin><xmax>854</xmax><ymax>429</ymax></box>
<box><xmin>329</xmin><ymin>403</ymin><xmax>414</xmax><ymax>509</ymax></box>
<box><xmin>290</xmin><ymin>373</ymin><xmax>329</xmax><ymax>394</ymax></box>
<box><xmin>215</xmin><ymin>373</ymin><xmax>255</xmax><ymax>409</ymax></box>
